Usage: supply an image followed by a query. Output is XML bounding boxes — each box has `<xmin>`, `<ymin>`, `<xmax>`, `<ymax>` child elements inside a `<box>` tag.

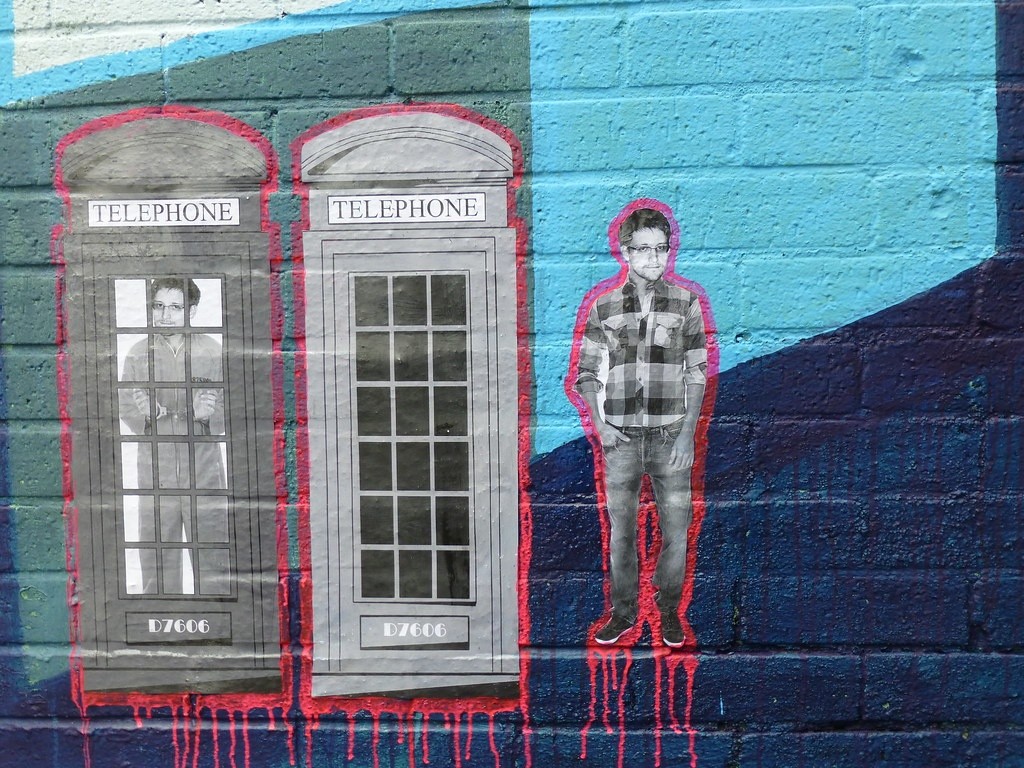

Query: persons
<box><xmin>118</xmin><ymin>278</ymin><xmax>230</xmax><ymax>594</ymax></box>
<box><xmin>572</xmin><ymin>209</ymin><xmax>708</xmax><ymax>648</ymax></box>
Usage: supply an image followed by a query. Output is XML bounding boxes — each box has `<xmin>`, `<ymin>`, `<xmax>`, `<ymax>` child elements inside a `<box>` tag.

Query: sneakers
<box><xmin>595</xmin><ymin>615</ymin><xmax>635</xmax><ymax>645</ymax></box>
<box><xmin>660</xmin><ymin>614</ymin><xmax>686</xmax><ymax>647</ymax></box>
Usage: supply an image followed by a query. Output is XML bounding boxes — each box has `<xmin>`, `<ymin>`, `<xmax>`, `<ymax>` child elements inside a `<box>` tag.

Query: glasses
<box><xmin>625</xmin><ymin>244</ymin><xmax>670</xmax><ymax>253</ymax></box>
<box><xmin>152</xmin><ymin>302</ymin><xmax>185</xmax><ymax>311</ymax></box>
